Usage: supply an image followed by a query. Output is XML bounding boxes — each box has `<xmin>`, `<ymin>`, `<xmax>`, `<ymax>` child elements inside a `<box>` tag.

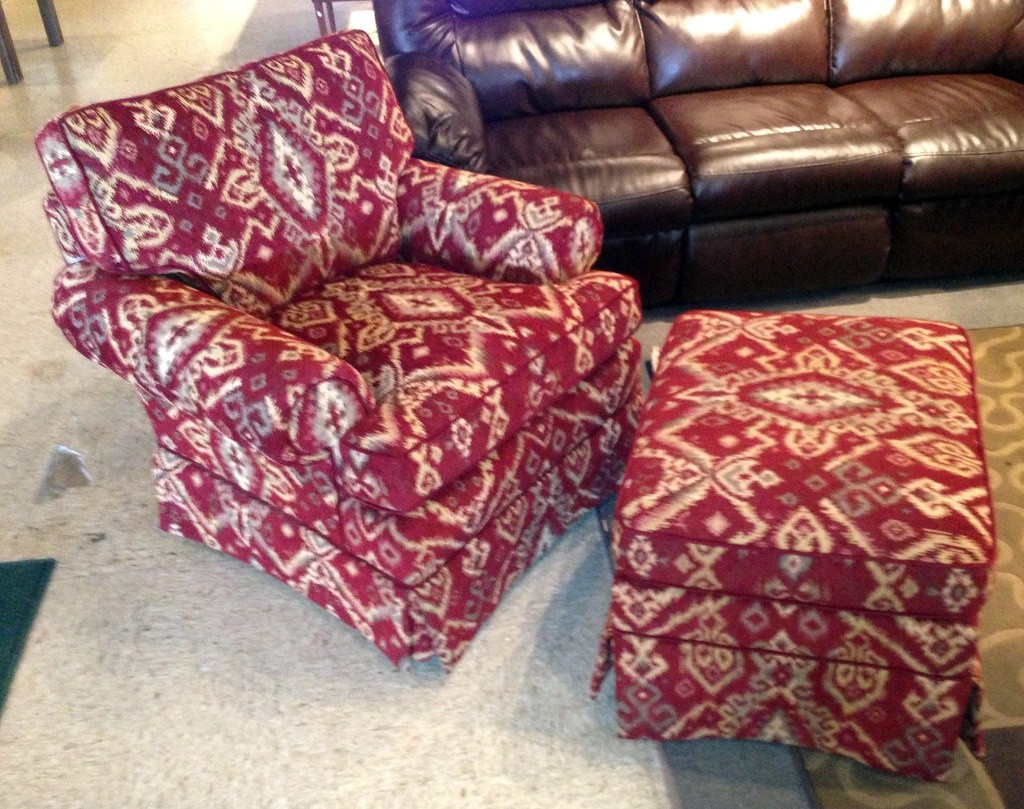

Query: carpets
<box><xmin>797</xmin><ymin>322</ymin><xmax>1023</xmax><ymax>808</ymax></box>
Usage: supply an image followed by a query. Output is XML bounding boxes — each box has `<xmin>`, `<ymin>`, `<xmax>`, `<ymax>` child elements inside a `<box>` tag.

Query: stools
<box><xmin>587</xmin><ymin>309</ymin><xmax>995</xmax><ymax>782</ymax></box>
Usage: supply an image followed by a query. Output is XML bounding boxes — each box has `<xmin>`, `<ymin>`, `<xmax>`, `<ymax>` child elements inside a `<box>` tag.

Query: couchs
<box><xmin>370</xmin><ymin>0</ymin><xmax>1024</xmax><ymax>307</ymax></box>
<box><xmin>33</xmin><ymin>29</ymin><xmax>646</xmax><ymax>677</ymax></box>
